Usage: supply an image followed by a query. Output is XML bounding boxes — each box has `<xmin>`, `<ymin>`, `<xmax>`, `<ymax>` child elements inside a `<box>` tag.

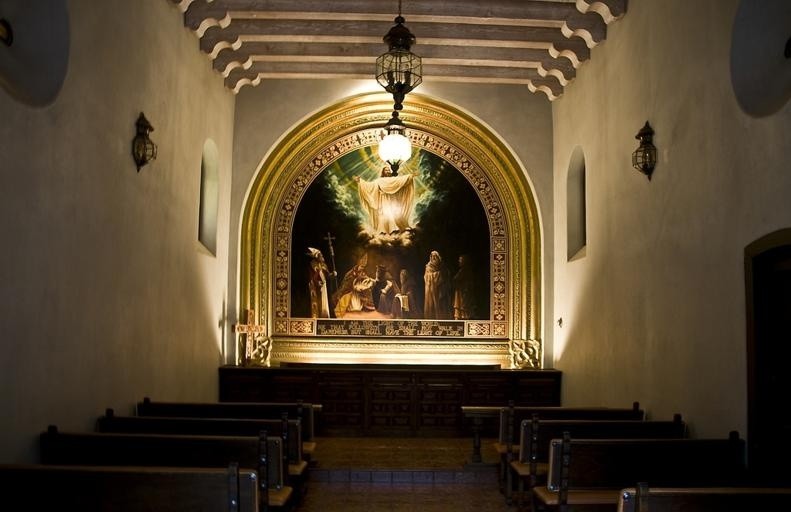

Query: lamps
<box><xmin>632</xmin><ymin>120</ymin><xmax>658</xmax><ymax>182</ymax></box>
<box><xmin>374</xmin><ymin>0</ymin><xmax>424</xmax><ymax>176</ymax></box>
<box><xmin>132</xmin><ymin>112</ymin><xmax>158</xmax><ymax>173</ymax></box>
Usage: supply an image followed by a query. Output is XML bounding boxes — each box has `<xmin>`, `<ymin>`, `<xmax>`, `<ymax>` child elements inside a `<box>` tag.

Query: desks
<box><xmin>218</xmin><ymin>361</ymin><xmax>562</xmax><ymax>438</ymax></box>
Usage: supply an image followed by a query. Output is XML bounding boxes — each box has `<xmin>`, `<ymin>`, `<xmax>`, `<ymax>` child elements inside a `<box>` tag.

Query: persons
<box><xmin>303</xmin><ymin>247</ymin><xmax>477</xmax><ymax>319</ymax></box>
<box><xmin>352</xmin><ymin>165</ymin><xmax>420</xmax><ymax>233</ymax></box>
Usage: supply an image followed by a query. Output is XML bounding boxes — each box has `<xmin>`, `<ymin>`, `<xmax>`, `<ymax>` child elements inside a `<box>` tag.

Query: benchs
<box><xmin>461</xmin><ymin>401</ymin><xmax>791</xmax><ymax>512</ymax></box>
<box><xmin>2</xmin><ymin>397</ymin><xmax>322</xmax><ymax>512</ymax></box>
